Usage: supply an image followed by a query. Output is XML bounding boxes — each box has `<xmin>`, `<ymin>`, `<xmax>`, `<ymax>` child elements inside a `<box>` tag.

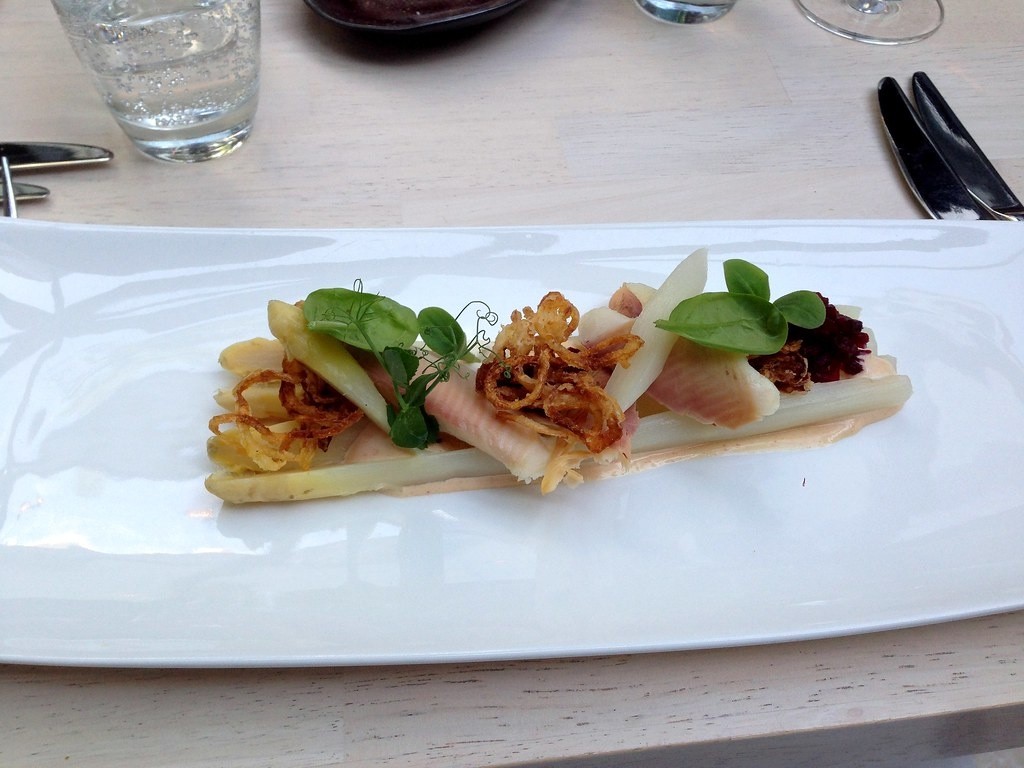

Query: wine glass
<box><xmin>794</xmin><ymin>0</ymin><xmax>944</xmax><ymax>46</ymax></box>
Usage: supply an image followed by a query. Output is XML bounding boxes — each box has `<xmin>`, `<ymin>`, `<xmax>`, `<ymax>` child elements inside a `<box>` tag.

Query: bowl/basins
<box><xmin>300</xmin><ymin>0</ymin><xmax>529</xmax><ymax>47</ymax></box>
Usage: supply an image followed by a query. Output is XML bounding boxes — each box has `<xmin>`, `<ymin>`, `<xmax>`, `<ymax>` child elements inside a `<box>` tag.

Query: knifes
<box><xmin>0</xmin><ymin>142</ymin><xmax>114</xmax><ymax>172</ymax></box>
<box><xmin>0</xmin><ymin>181</ymin><xmax>50</xmax><ymax>202</ymax></box>
<box><xmin>878</xmin><ymin>77</ymin><xmax>986</xmax><ymax>221</ymax></box>
<box><xmin>911</xmin><ymin>70</ymin><xmax>1024</xmax><ymax>221</ymax></box>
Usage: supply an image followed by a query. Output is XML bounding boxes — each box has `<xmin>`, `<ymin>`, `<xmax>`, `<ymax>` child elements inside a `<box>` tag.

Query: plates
<box><xmin>0</xmin><ymin>216</ymin><xmax>1023</xmax><ymax>668</ymax></box>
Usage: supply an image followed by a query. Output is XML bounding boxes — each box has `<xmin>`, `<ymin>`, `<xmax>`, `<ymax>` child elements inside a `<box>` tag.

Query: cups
<box><xmin>50</xmin><ymin>0</ymin><xmax>260</xmax><ymax>164</ymax></box>
<box><xmin>632</xmin><ymin>0</ymin><xmax>738</xmax><ymax>24</ymax></box>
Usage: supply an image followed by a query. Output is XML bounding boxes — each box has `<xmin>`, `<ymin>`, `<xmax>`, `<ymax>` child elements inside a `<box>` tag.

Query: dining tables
<box><xmin>0</xmin><ymin>0</ymin><xmax>1024</xmax><ymax>767</ymax></box>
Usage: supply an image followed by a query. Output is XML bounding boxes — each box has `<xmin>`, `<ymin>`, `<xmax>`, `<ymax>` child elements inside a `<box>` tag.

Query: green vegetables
<box><xmin>652</xmin><ymin>258</ymin><xmax>827</xmax><ymax>354</ymax></box>
<box><xmin>300</xmin><ymin>282</ymin><xmax>512</xmax><ymax>451</ymax></box>
